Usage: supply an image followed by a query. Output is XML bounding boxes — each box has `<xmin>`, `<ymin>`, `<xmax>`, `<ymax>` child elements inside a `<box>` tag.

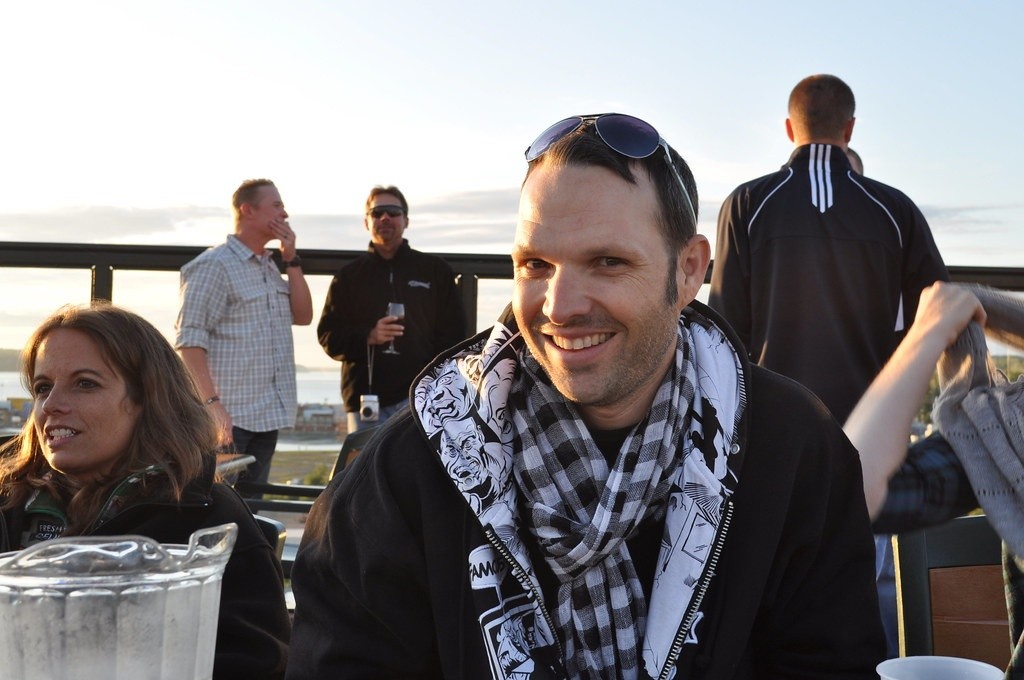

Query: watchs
<box><xmin>283</xmin><ymin>256</ymin><xmax>300</xmax><ymax>267</ymax></box>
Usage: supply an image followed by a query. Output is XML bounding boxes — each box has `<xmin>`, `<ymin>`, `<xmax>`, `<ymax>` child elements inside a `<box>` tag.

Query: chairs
<box><xmin>893</xmin><ymin>516</ymin><xmax>1013</xmax><ymax>673</ymax></box>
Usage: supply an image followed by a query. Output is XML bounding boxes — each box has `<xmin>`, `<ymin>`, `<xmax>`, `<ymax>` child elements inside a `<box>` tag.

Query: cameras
<box><xmin>360</xmin><ymin>394</ymin><xmax>379</xmax><ymax>422</ymax></box>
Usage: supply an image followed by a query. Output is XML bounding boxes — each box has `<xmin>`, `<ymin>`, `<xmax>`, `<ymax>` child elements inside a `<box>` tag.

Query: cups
<box><xmin>875</xmin><ymin>655</ymin><xmax>1005</xmax><ymax>680</ymax></box>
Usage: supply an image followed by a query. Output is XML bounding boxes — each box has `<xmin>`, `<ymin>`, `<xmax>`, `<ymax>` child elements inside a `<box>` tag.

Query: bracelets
<box><xmin>206</xmin><ymin>396</ymin><xmax>220</xmax><ymax>404</ymax></box>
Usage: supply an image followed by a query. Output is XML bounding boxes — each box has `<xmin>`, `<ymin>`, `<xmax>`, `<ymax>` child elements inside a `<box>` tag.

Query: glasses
<box><xmin>367</xmin><ymin>204</ymin><xmax>403</xmax><ymax>219</ymax></box>
<box><xmin>525</xmin><ymin>112</ymin><xmax>696</xmax><ymax>237</ymax></box>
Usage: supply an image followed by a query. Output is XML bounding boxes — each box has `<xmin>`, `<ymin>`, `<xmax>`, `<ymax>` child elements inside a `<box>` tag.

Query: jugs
<box><xmin>0</xmin><ymin>523</ymin><xmax>239</xmax><ymax>680</ymax></box>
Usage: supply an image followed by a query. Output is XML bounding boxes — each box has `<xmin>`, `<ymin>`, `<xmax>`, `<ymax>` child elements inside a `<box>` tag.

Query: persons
<box><xmin>174</xmin><ymin>179</ymin><xmax>313</xmax><ymax>515</ymax></box>
<box><xmin>708</xmin><ymin>74</ymin><xmax>950</xmax><ymax>658</ymax></box>
<box><xmin>0</xmin><ymin>308</ymin><xmax>293</xmax><ymax>680</ymax></box>
<box><xmin>841</xmin><ymin>281</ymin><xmax>1024</xmax><ymax>680</ymax></box>
<box><xmin>292</xmin><ymin>114</ymin><xmax>889</xmax><ymax>680</ymax></box>
<box><xmin>318</xmin><ymin>188</ymin><xmax>466</xmax><ymax>435</ymax></box>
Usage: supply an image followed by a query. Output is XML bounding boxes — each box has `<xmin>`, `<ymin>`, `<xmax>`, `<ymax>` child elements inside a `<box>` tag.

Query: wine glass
<box><xmin>382</xmin><ymin>302</ymin><xmax>405</xmax><ymax>355</ymax></box>
